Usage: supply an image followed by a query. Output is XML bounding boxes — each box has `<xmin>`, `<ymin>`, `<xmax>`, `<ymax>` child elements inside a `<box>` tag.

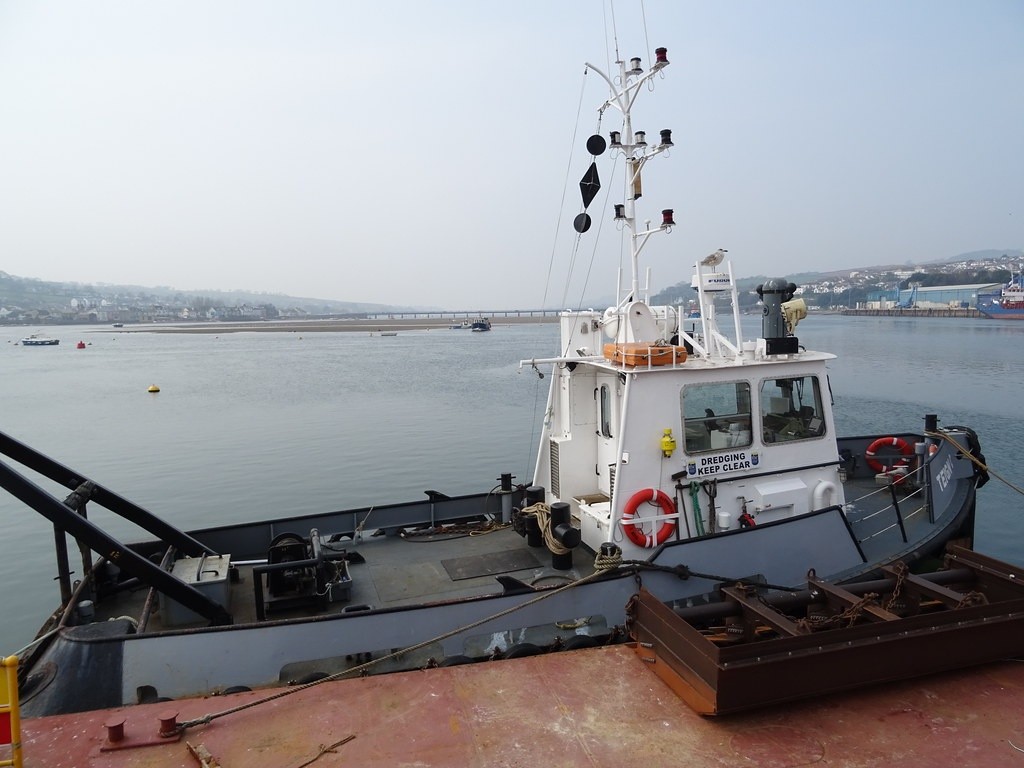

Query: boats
<box><xmin>471</xmin><ymin>315</ymin><xmax>492</xmax><ymax>332</ymax></box>
<box><xmin>148</xmin><ymin>383</ymin><xmax>161</xmax><ymax>393</ymax></box>
<box><xmin>21</xmin><ymin>330</ymin><xmax>61</xmax><ymax>346</ymax></box>
<box><xmin>975</xmin><ymin>268</ymin><xmax>1024</xmax><ymax>320</ymax></box>
<box><xmin>381</xmin><ymin>332</ymin><xmax>398</xmax><ymax>337</ymax></box>
<box><xmin>76</xmin><ymin>341</ymin><xmax>86</xmax><ymax>349</ymax></box>
<box><xmin>448</xmin><ymin>319</ymin><xmax>472</xmax><ymax>330</ymax></box>
<box><xmin>0</xmin><ymin>0</ymin><xmax>992</xmax><ymax>719</ymax></box>
<box><xmin>112</xmin><ymin>323</ymin><xmax>124</xmax><ymax>328</ymax></box>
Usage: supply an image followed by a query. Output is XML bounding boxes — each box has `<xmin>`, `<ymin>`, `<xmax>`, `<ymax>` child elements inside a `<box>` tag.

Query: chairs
<box><xmin>704</xmin><ymin>409</ymin><xmax>721</xmax><ymax>435</ymax></box>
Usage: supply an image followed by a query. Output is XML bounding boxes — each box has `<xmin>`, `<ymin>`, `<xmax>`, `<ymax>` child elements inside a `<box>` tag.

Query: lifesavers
<box><xmin>623</xmin><ymin>488</ymin><xmax>678</xmax><ymax>549</ymax></box>
<box><xmin>929</xmin><ymin>443</ymin><xmax>938</xmax><ymax>457</ymax></box>
<box><xmin>866</xmin><ymin>437</ymin><xmax>914</xmax><ymax>473</ymax></box>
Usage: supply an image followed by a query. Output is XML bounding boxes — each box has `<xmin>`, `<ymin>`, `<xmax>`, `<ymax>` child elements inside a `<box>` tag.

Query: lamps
<box><xmin>780</xmin><ymin>297</ymin><xmax>808</xmax><ymax>352</ymax></box>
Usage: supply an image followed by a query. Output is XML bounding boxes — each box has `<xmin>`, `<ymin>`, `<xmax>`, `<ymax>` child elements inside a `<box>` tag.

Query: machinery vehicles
<box><xmin>892</xmin><ymin>282</ymin><xmax>919</xmax><ymax>309</ymax></box>
